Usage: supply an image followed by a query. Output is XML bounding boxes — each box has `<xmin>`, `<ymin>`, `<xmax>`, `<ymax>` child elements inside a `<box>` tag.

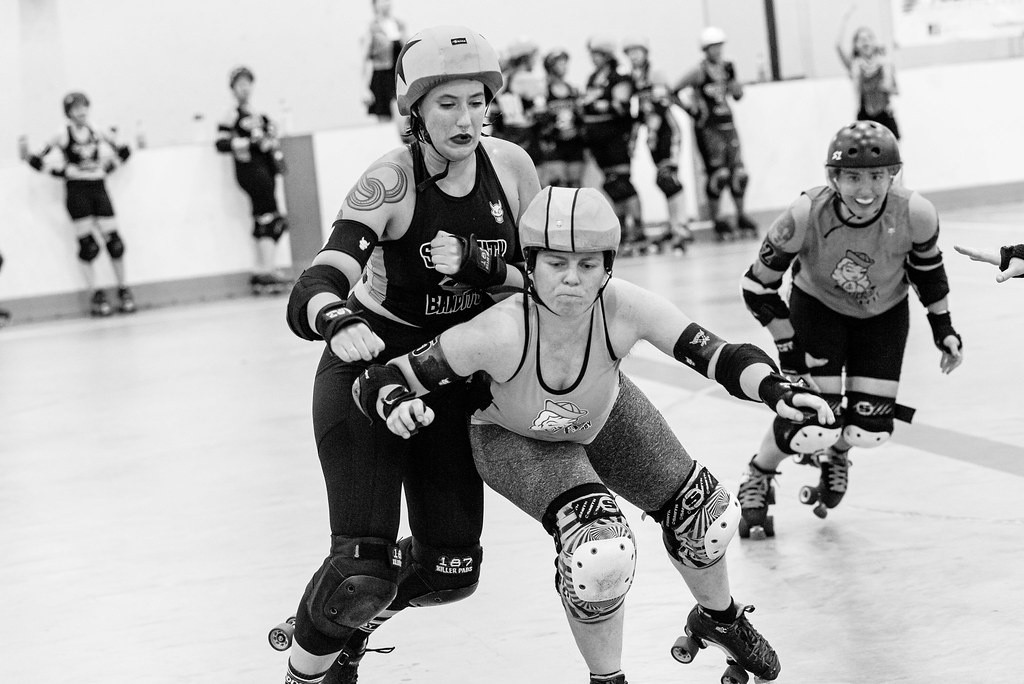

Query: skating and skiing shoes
<box><xmin>734</xmin><ymin>453</ymin><xmax>776</xmax><ymax>538</ymax></box>
<box><xmin>119</xmin><ymin>289</ymin><xmax>138</xmax><ymax>313</ymax></box>
<box><xmin>267</xmin><ymin>612</ymin><xmax>369</xmax><ymax>684</ymax></box>
<box><xmin>713</xmin><ymin>221</ymin><xmax>738</xmax><ymax>240</ymax></box>
<box><xmin>621</xmin><ymin>219</ymin><xmax>696</xmax><ymax>258</ymax></box>
<box><xmin>91</xmin><ymin>291</ymin><xmax>113</xmax><ymax>315</ymax></box>
<box><xmin>792</xmin><ymin>448</ymin><xmax>848</xmax><ymax>519</ymax></box>
<box><xmin>671</xmin><ymin>602</ymin><xmax>781</xmax><ymax>684</ymax></box>
<box><xmin>248</xmin><ymin>278</ymin><xmax>287</xmax><ymax>294</ymax></box>
<box><xmin>734</xmin><ymin>219</ymin><xmax>759</xmax><ymax>236</ymax></box>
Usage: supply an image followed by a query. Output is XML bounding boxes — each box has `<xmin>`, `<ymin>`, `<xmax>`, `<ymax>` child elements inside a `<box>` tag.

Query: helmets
<box><xmin>506</xmin><ymin>45</ymin><xmax>538</xmax><ymax>70</ymax></box>
<box><xmin>622</xmin><ymin>36</ymin><xmax>649</xmax><ymax>51</ymax></box>
<box><xmin>394</xmin><ymin>24</ymin><xmax>503</xmax><ymax>116</ymax></box>
<box><xmin>825</xmin><ymin>121</ymin><xmax>903</xmax><ymax>168</ymax></box>
<box><xmin>518</xmin><ymin>186</ymin><xmax>621</xmax><ymax>273</ymax></box>
<box><xmin>589</xmin><ymin>37</ymin><xmax>616</xmax><ymax>53</ymax></box>
<box><xmin>544</xmin><ymin>52</ymin><xmax>570</xmax><ymax>67</ymax></box>
<box><xmin>229</xmin><ymin>67</ymin><xmax>254</xmax><ymax>86</ymax></box>
<box><xmin>63</xmin><ymin>93</ymin><xmax>90</xmax><ymax>117</ymax></box>
<box><xmin>699</xmin><ymin>27</ymin><xmax>725</xmax><ymax>49</ymax></box>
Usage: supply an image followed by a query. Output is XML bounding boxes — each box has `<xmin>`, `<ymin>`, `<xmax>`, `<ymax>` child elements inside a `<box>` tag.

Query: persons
<box><xmin>736</xmin><ymin>125</ymin><xmax>963</xmax><ymax>539</ymax></box>
<box><xmin>834</xmin><ymin>0</ymin><xmax>900</xmax><ymax>141</ymax></box>
<box><xmin>354</xmin><ymin>184</ymin><xmax>835</xmax><ymax>684</ymax></box>
<box><xmin>267</xmin><ymin>29</ymin><xmax>541</xmax><ymax>684</ymax></box>
<box><xmin>26</xmin><ymin>92</ymin><xmax>135</xmax><ymax>315</ymax></box>
<box><xmin>481</xmin><ymin>25</ymin><xmax>759</xmax><ymax>258</ymax></box>
<box><xmin>954</xmin><ymin>243</ymin><xmax>1024</xmax><ymax>283</ymax></box>
<box><xmin>358</xmin><ymin>0</ymin><xmax>408</xmax><ymax>122</ymax></box>
<box><xmin>216</xmin><ymin>65</ymin><xmax>291</xmax><ymax>297</ymax></box>
